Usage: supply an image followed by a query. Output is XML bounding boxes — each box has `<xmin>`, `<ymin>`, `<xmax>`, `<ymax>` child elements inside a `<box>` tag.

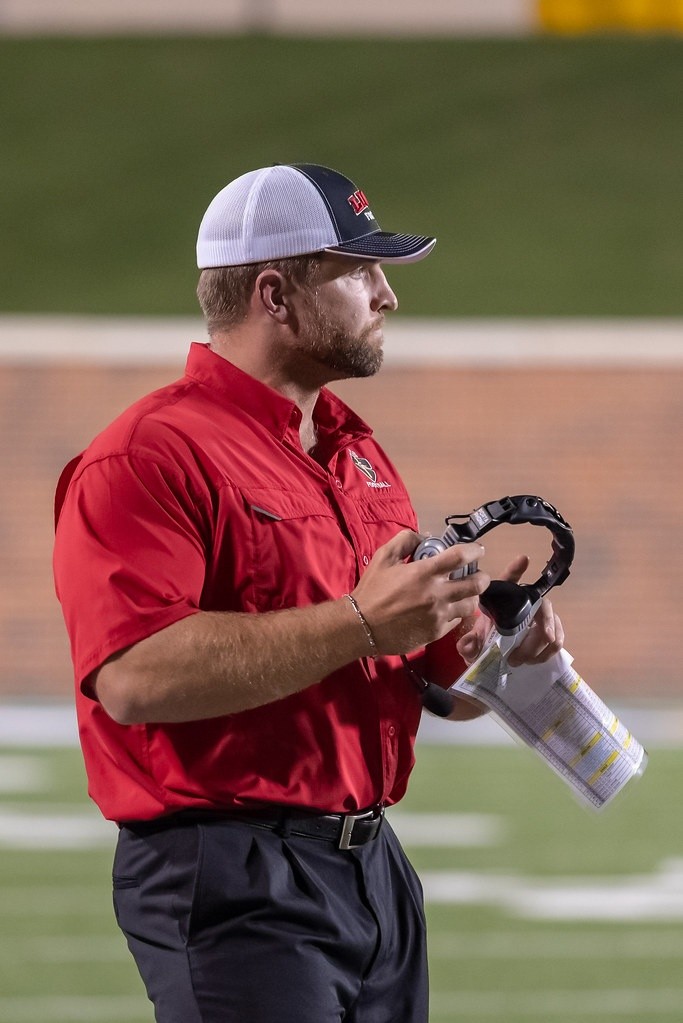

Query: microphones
<box><xmin>400</xmin><ymin>654</ymin><xmax>455</xmax><ymax>720</ymax></box>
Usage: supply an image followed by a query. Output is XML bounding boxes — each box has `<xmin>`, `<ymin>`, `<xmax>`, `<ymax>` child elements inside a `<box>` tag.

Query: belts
<box><xmin>184</xmin><ymin>807</ymin><xmax>385</xmax><ymax>849</ymax></box>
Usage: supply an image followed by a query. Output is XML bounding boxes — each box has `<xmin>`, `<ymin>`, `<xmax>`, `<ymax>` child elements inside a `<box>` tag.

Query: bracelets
<box><xmin>342</xmin><ymin>594</ymin><xmax>379</xmax><ymax>662</ymax></box>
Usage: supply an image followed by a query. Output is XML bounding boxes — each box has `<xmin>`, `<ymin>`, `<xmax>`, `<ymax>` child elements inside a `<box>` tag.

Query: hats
<box><xmin>196</xmin><ymin>162</ymin><xmax>436</xmax><ymax>270</ymax></box>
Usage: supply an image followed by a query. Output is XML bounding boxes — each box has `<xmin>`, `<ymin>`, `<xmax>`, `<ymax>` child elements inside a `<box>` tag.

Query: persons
<box><xmin>54</xmin><ymin>162</ymin><xmax>566</xmax><ymax>1023</ymax></box>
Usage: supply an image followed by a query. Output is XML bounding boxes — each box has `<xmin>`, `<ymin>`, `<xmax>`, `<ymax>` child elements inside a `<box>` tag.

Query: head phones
<box><xmin>406</xmin><ymin>495</ymin><xmax>576</xmax><ymax>635</ymax></box>
